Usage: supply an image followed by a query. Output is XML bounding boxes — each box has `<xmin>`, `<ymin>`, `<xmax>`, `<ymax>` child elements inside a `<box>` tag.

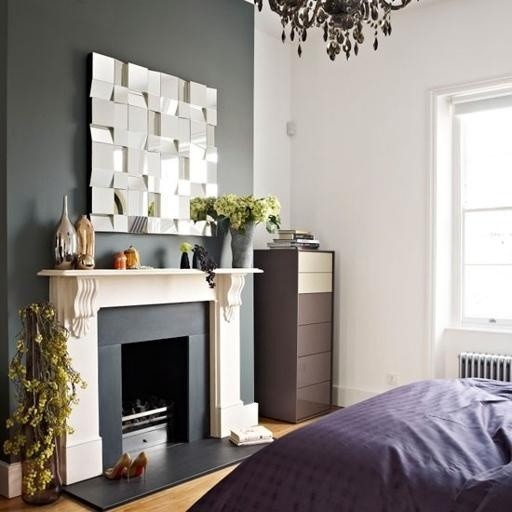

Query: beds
<box><xmin>185</xmin><ymin>378</ymin><xmax>512</xmax><ymax>512</ymax></box>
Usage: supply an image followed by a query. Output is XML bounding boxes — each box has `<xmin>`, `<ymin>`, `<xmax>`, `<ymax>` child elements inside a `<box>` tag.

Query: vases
<box><xmin>21</xmin><ymin>403</ymin><xmax>62</xmax><ymax>505</ymax></box>
<box><xmin>193</xmin><ymin>254</ymin><xmax>201</xmax><ymax>268</ymax></box>
<box><xmin>230</xmin><ymin>219</ymin><xmax>253</xmax><ymax>268</ymax></box>
<box><xmin>181</xmin><ymin>252</ymin><xmax>190</xmax><ymax>268</ymax></box>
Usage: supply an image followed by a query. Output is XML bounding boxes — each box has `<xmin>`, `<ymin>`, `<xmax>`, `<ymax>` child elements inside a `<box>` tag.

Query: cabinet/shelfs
<box><xmin>253</xmin><ymin>249</ymin><xmax>334</xmax><ymax>424</ymax></box>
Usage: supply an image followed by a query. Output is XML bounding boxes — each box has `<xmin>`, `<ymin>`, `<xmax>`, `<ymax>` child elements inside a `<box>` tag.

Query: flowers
<box><xmin>213</xmin><ymin>195</ymin><xmax>282</xmax><ymax>236</ymax></box>
<box><xmin>190</xmin><ymin>196</ymin><xmax>216</xmax><ymax>225</ymax></box>
<box><xmin>0</xmin><ymin>301</ymin><xmax>90</xmax><ymax>497</ymax></box>
<box><xmin>191</xmin><ymin>243</ymin><xmax>217</xmax><ymax>288</ymax></box>
<box><xmin>179</xmin><ymin>242</ymin><xmax>193</xmax><ymax>252</ymax></box>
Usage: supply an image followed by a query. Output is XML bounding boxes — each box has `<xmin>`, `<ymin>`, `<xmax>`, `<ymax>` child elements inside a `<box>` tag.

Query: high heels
<box><xmin>122</xmin><ymin>452</ymin><xmax>149</xmax><ymax>484</ymax></box>
<box><xmin>105</xmin><ymin>452</ymin><xmax>132</xmax><ymax>481</ymax></box>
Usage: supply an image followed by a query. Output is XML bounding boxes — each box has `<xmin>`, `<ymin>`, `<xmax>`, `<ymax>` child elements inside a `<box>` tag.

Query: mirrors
<box><xmin>91</xmin><ymin>52</ymin><xmax>218</xmax><ymax>238</ymax></box>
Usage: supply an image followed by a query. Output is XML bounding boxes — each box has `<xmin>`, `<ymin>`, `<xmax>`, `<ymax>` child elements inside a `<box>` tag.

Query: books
<box><xmin>266</xmin><ymin>229</ymin><xmax>320</xmax><ymax>249</ymax></box>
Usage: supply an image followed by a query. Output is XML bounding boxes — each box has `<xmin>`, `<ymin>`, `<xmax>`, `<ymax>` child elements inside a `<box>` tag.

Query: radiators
<box><xmin>457</xmin><ymin>353</ymin><xmax>512</xmax><ymax>382</ymax></box>
